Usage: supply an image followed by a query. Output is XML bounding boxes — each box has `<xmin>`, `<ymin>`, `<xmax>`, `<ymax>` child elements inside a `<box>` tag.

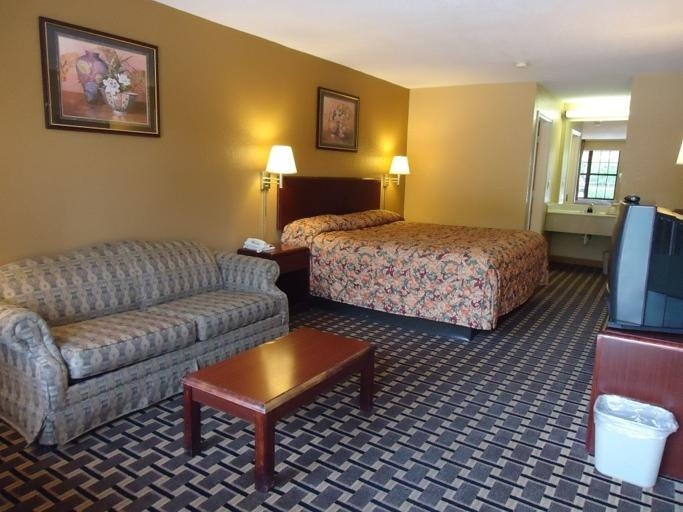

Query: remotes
<box><xmin>623</xmin><ymin>195</ymin><xmax>641</xmax><ymax>204</ymax></box>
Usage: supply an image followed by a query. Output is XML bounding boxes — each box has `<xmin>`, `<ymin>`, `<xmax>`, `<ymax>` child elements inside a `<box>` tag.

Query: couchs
<box><xmin>0</xmin><ymin>238</ymin><xmax>289</xmax><ymax>445</ymax></box>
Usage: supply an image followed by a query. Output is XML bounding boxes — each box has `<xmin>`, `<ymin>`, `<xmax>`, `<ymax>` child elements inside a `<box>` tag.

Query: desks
<box><xmin>584</xmin><ymin>327</ymin><xmax>683</xmax><ymax>483</ymax></box>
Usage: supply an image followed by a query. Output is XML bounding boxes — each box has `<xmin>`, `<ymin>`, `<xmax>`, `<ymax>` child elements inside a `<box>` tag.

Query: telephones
<box><xmin>243</xmin><ymin>238</ymin><xmax>275</xmax><ymax>253</ymax></box>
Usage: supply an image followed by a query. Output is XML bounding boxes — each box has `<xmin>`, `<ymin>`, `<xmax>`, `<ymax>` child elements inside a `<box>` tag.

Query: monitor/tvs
<box><xmin>606</xmin><ymin>202</ymin><xmax>683</xmax><ymax>334</ymax></box>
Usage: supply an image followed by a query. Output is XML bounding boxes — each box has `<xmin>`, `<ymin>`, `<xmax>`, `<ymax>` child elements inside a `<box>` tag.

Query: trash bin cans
<box><xmin>593</xmin><ymin>394</ymin><xmax>674</xmax><ymax>488</ymax></box>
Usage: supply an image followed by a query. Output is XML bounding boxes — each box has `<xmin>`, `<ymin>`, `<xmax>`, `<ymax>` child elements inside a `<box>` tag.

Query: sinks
<box><xmin>567</xmin><ymin>211</ymin><xmax>605</xmax><ymax>216</ymax></box>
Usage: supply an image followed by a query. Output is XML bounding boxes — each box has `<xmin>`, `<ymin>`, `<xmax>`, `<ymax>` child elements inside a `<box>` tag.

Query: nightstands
<box><xmin>236</xmin><ymin>243</ymin><xmax>310</xmax><ymax>315</ymax></box>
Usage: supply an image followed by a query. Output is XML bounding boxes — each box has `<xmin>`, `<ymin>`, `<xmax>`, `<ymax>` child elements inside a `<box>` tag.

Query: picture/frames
<box><xmin>37</xmin><ymin>14</ymin><xmax>160</xmax><ymax>137</ymax></box>
<box><xmin>316</xmin><ymin>86</ymin><xmax>359</xmax><ymax>152</ymax></box>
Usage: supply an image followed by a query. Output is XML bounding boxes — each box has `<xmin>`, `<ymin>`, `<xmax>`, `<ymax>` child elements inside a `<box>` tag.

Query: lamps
<box><xmin>382</xmin><ymin>156</ymin><xmax>410</xmax><ymax>210</ymax></box>
<box><xmin>261</xmin><ymin>145</ymin><xmax>296</xmax><ymax>240</ymax></box>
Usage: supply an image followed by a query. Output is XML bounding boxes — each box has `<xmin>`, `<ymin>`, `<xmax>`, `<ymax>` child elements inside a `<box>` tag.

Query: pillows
<box><xmin>281</xmin><ymin>209</ymin><xmax>353</xmax><ymax>237</ymax></box>
<box><xmin>338</xmin><ymin>208</ymin><xmax>405</xmax><ymax>231</ymax></box>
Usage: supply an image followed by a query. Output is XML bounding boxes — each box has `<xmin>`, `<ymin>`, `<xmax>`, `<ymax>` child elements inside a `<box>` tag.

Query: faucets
<box><xmin>589</xmin><ymin>203</ymin><xmax>594</xmax><ymax>206</ymax></box>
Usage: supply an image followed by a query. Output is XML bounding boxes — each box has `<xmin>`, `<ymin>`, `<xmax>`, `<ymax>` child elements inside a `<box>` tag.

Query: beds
<box><xmin>276</xmin><ymin>175</ymin><xmax>548</xmax><ymax>342</ymax></box>
